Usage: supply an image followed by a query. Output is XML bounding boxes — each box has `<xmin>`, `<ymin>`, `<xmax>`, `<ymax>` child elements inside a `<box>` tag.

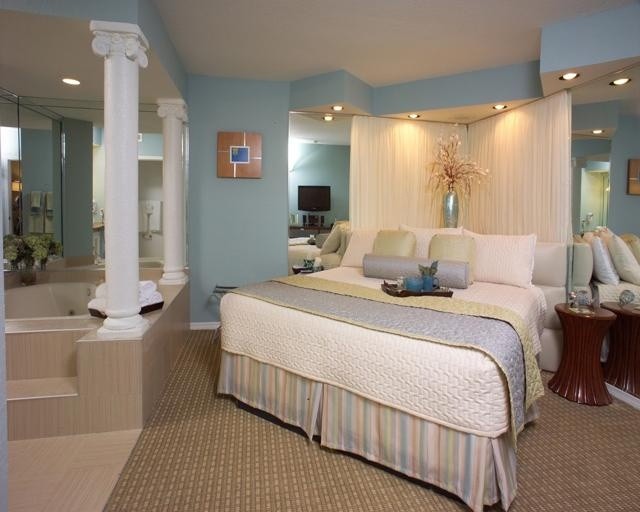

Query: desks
<box><xmin>547</xmin><ymin>302</ymin><xmax>618</xmax><ymax>406</ymax></box>
<box><xmin>290</xmin><ymin>226</ymin><xmax>331</xmax><ymax>233</ymax></box>
<box><xmin>600</xmin><ymin>300</ymin><xmax>640</xmax><ymax>400</ymax></box>
<box><xmin>292</xmin><ymin>264</ymin><xmax>324</xmax><ymax>274</ymax></box>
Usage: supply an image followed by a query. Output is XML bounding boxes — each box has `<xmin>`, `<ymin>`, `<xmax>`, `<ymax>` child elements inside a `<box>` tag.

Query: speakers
<box><xmin>303</xmin><ymin>215</ymin><xmax>306</xmax><ymax>226</ymax></box>
<box><xmin>321</xmin><ymin>216</ymin><xmax>324</xmax><ymax>227</ymax></box>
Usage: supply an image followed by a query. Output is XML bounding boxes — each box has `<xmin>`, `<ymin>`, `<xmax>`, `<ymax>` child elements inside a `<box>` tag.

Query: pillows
<box><xmin>340</xmin><ymin>222</ymin><xmax>536</xmax><ymax>289</ymax></box>
<box><xmin>583</xmin><ymin>225</ymin><xmax>640</xmax><ymax>286</ymax></box>
<box><xmin>316</xmin><ymin>220</ymin><xmax>348</xmax><ymax>255</ymax></box>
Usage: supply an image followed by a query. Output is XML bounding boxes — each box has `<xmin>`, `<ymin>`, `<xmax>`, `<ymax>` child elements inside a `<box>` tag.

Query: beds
<box><xmin>573</xmin><ymin>234</ymin><xmax>640</xmax><ymax>304</ymax></box>
<box><xmin>288</xmin><ymin>236</ymin><xmax>351</xmax><ymax>275</ymax></box>
<box><xmin>217</xmin><ymin>242</ymin><xmax>569</xmax><ymax>512</ymax></box>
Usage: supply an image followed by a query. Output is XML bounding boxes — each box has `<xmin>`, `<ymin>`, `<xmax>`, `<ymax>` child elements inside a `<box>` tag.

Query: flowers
<box><xmin>23</xmin><ymin>234</ymin><xmax>64</xmax><ymax>263</ymax></box>
<box><xmin>420</xmin><ymin>128</ymin><xmax>490</xmax><ymax>227</ymax></box>
<box><xmin>3</xmin><ymin>233</ymin><xmax>26</xmax><ymax>265</ymax></box>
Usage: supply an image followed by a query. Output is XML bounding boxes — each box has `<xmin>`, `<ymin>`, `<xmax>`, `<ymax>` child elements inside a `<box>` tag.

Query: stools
<box><xmin>207</xmin><ymin>281</ymin><xmax>239</xmax><ymax>343</ymax></box>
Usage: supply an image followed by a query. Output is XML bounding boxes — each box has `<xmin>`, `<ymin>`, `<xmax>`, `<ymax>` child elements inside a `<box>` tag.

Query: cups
<box><xmin>618</xmin><ymin>289</ymin><xmax>634</xmax><ymax>305</ymax></box>
<box><xmin>406</xmin><ymin>277</ymin><xmax>423</xmax><ymax>292</ymax></box>
<box><xmin>424</xmin><ymin>274</ymin><xmax>439</xmax><ymax>291</ymax></box>
<box><xmin>570</xmin><ymin>289</ymin><xmax>596</xmax><ymax>314</ymax></box>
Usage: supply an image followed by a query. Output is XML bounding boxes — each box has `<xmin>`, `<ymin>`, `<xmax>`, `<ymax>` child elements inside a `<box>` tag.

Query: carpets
<box><xmin>103</xmin><ymin>328</ymin><xmax>640</xmax><ymax>512</ymax></box>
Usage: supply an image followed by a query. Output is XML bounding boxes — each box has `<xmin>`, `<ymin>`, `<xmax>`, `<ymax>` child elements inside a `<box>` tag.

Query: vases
<box><xmin>41</xmin><ymin>261</ymin><xmax>46</xmax><ymax>271</ymax></box>
<box><xmin>443</xmin><ymin>189</ymin><xmax>458</xmax><ymax>229</ymax></box>
<box><xmin>11</xmin><ymin>262</ymin><xmax>17</xmax><ymax>270</ymax></box>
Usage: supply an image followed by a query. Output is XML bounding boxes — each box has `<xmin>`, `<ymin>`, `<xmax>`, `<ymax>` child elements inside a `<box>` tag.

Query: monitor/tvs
<box><xmin>298</xmin><ymin>186</ymin><xmax>330</xmax><ymax>211</ymax></box>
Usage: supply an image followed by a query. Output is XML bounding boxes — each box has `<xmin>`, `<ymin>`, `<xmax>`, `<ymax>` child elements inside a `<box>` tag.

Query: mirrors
<box><xmin>18</xmin><ymin>95</ymin><xmax>189</xmax><ymax>269</ymax></box>
<box><xmin>569</xmin><ymin>62</ymin><xmax>640</xmax><ymax>410</ymax></box>
<box><xmin>0</xmin><ymin>87</ymin><xmax>62</xmax><ymax>272</ymax></box>
<box><xmin>288</xmin><ymin>110</ymin><xmax>352</xmax><ymax>275</ymax></box>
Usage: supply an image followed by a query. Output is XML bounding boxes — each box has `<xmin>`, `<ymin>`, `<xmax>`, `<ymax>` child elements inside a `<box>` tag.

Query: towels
<box><xmin>30</xmin><ymin>190</ymin><xmax>43</xmax><ymax>234</ymax></box>
<box><xmin>46</xmin><ymin>191</ymin><xmax>56</xmax><ymax>235</ymax></box>
<box><xmin>87</xmin><ymin>279</ymin><xmax>164</xmax><ymax>315</ymax></box>
